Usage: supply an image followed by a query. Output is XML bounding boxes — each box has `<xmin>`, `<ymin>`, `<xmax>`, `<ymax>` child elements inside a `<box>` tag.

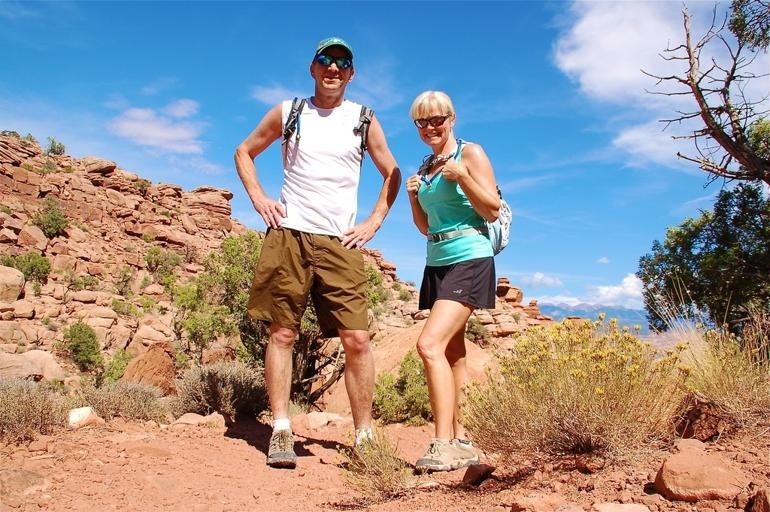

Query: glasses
<box><xmin>316</xmin><ymin>54</ymin><xmax>352</xmax><ymax>69</ymax></box>
<box><xmin>414</xmin><ymin>115</ymin><xmax>449</xmax><ymax>128</ymax></box>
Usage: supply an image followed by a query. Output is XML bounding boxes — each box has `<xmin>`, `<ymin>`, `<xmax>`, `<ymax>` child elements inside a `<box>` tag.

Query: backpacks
<box><xmin>486</xmin><ymin>184</ymin><xmax>512</xmax><ymax>255</ymax></box>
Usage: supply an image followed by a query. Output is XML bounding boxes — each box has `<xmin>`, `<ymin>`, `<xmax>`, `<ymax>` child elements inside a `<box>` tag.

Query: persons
<box><xmin>234</xmin><ymin>37</ymin><xmax>402</xmax><ymax>474</ymax></box>
<box><xmin>406</xmin><ymin>90</ymin><xmax>513</xmax><ymax>471</ymax></box>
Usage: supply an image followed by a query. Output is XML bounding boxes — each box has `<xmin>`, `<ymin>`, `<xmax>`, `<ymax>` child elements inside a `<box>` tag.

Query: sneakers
<box><xmin>416</xmin><ymin>439</ymin><xmax>479</xmax><ymax>472</ymax></box>
<box><xmin>267</xmin><ymin>429</ymin><xmax>296</xmax><ymax>467</ymax></box>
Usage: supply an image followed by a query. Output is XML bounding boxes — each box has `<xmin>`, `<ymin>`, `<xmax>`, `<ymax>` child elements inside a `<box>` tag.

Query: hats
<box><xmin>312</xmin><ymin>38</ymin><xmax>353</xmax><ymax>62</ymax></box>
